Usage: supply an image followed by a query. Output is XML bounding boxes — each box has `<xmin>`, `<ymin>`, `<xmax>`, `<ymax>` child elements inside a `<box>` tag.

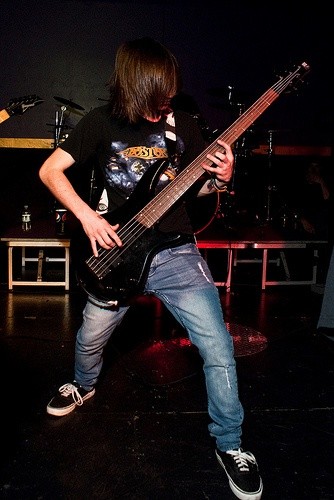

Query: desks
<box><xmin>1</xmin><ymin>229</ymin><xmax>328</xmax><ymax>292</ymax></box>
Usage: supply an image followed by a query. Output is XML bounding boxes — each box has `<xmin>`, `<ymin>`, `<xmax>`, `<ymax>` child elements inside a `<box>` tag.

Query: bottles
<box><xmin>21</xmin><ymin>205</ymin><xmax>31</xmax><ymax>233</ymax></box>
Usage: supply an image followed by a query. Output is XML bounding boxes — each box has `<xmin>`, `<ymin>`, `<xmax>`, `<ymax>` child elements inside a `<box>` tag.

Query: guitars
<box><xmin>0</xmin><ymin>93</ymin><xmax>47</xmax><ymax>124</ymax></box>
<box><xmin>69</xmin><ymin>56</ymin><xmax>317</xmax><ymax>302</ymax></box>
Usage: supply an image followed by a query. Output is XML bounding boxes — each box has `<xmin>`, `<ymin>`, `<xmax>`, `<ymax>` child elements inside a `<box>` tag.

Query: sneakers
<box><xmin>215</xmin><ymin>448</ymin><xmax>263</xmax><ymax>500</ymax></box>
<box><xmin>47</xmin><ymin>381</ymin><xmax>96</xmax><ymax>417</ymax></box>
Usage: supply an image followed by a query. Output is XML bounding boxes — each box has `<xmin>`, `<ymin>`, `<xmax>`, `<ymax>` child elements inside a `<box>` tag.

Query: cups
<box><xmin>54</xmin><ymin>209</ymin><xmax>68</xmax><ymax>235</ymax></box>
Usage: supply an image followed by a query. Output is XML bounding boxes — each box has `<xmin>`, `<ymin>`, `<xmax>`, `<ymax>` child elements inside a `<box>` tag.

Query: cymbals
<box><xmin>52</xmin><ymin>95</ymin><xmax>86</xmax><ymax>111</ymax></box>
<box><xmin>204</xmin><ymin>84</ymin><xmax>254</xmax><ymax>100</ymax></box>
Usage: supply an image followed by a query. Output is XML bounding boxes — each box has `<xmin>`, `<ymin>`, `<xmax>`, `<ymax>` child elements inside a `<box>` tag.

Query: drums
<box><xmin>181</xmin><ymin>169</ymin><xmax>221</xmax><ymax>235</ymax></box>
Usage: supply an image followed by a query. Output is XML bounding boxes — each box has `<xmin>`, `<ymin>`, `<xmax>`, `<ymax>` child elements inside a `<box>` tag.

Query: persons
<box><xmin>39</xmin><ymin>35</ymin><xmax>263</xmax><ymax>500</ymax></box>
<box><xmin>169</xmin><ymin>75</ymin><xmax>200</xmax><ymax>115</ymax></box>
<box><xmin>286</xmin><ymin>155</ymin><xmax>334</xmax><ymax>241</ymax></box>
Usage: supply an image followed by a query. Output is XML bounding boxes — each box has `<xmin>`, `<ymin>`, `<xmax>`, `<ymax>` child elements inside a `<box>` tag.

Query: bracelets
<box><xmin>208</xmin><ymin>178</ymin><xmax>221</xmax><ymax>191</ymax></box>
